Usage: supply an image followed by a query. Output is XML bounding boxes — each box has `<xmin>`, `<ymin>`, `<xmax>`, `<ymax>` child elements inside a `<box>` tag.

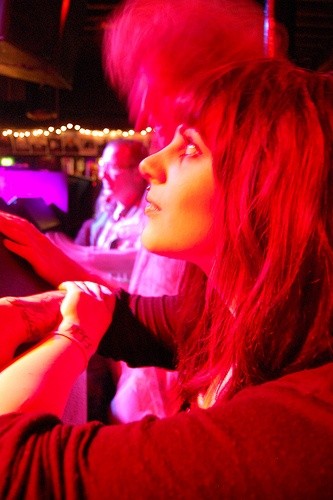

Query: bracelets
<box><xmin>52</xmin><ymin>322</ymin><xmax>96</xmax><ymax>357</ymax></box>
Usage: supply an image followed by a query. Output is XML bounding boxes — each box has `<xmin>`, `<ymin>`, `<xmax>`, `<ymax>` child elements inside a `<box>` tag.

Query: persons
<box><xmin>0</xmin><ymin>55</ymin><xmax>333</xmax><ymax>500</ymax></box>
<box><xmin>85</xmin><ymin>137</ymin><xmax>150</xmax><ymax>250</ymax></box>
